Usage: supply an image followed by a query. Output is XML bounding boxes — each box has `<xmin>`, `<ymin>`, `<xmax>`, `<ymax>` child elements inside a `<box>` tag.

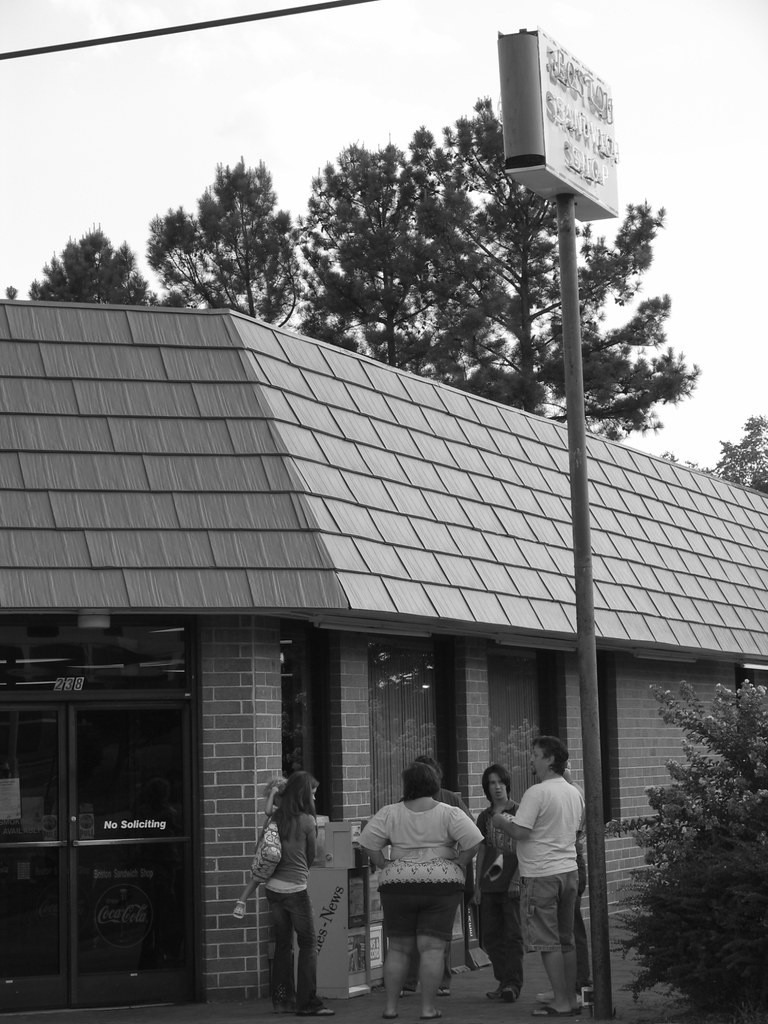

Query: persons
<box><xmin>493</xmin><ymin>735</ymin><xmax>593</xmax><ymax>1015</ymax></box>
<box><xmin>472</xmin><ymin>763</ymin><xmax>525</xmax><ymax>1001</ymax></box>
<box><xmin>357</xmin><ymin>756</ymin><xmax>485</xmax><ymax>1019</ymax></box>
<box><xmin>232</xmin><ymin>770</ymin><xmax>335</xmax><ymax>1015</ymax></box>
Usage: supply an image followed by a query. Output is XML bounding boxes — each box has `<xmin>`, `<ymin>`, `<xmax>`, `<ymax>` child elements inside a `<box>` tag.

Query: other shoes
<box><xmin>502</xmin><ymin>987</ymin><xmax>516</xmax><ymax>1001</ymax></box>
<box><xmin>485</xmin><ymin>987</ymin><xmax>502</xmax><ymax>999</ymax></box>
<box><xmin>536</xmin><ymin>990</ymin><xmax>558</xmax><ymax>1002</ymax></box>
<box><xmin>576</xmin><ymin>994</ymin><xmax>594</xmax><ymax>1008</ymax></box>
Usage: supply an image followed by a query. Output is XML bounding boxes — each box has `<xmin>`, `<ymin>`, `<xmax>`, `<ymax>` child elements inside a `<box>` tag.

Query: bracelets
<box><xmin>510</xmin><ymin>880</ymin><xmax>520</xmax><ymax>885</ymax></box>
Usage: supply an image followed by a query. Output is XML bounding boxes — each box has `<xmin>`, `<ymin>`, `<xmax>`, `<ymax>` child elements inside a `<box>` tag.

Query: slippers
<box><xmin>533</xmin><ymin>1006</ymin><xmax>580</xmax><ymax>1016</ymax></box>
<box><xmin>420</xmin><ymin>1008</ymin><xmax>441</xmax><ymax>1018</ymax></box>
<box><xmin>383</xmin><ymin>1010</ymin><xmax>398</xmax><ymax>1018</ymax></box>
<box><xmin>437</xmin><ymin>986</ymin><xmax>451</xmax><ymax>996</ymax></box>
<box><xmin>297</xmin><ymin>1007</ymin><xmax>334</xmax><ymax>1015</ymax></box>
<box><xmin>398</xmin><ymin>989</ymin><xmax>414</xmax><ymax>997</ymax></box>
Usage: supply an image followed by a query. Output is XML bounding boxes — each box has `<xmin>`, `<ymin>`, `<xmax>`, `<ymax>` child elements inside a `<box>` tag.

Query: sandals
<box><xmin>234</xmin><ymin>902</ymin><xmax>247</xmax><ymax>919</ymax></box>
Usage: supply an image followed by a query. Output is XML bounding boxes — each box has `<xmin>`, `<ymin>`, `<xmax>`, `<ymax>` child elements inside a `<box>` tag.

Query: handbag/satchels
<box><xmin>251</xmin><ymin>815</ymin><xmax>282</xmax><ymax>882</ymax></box>
